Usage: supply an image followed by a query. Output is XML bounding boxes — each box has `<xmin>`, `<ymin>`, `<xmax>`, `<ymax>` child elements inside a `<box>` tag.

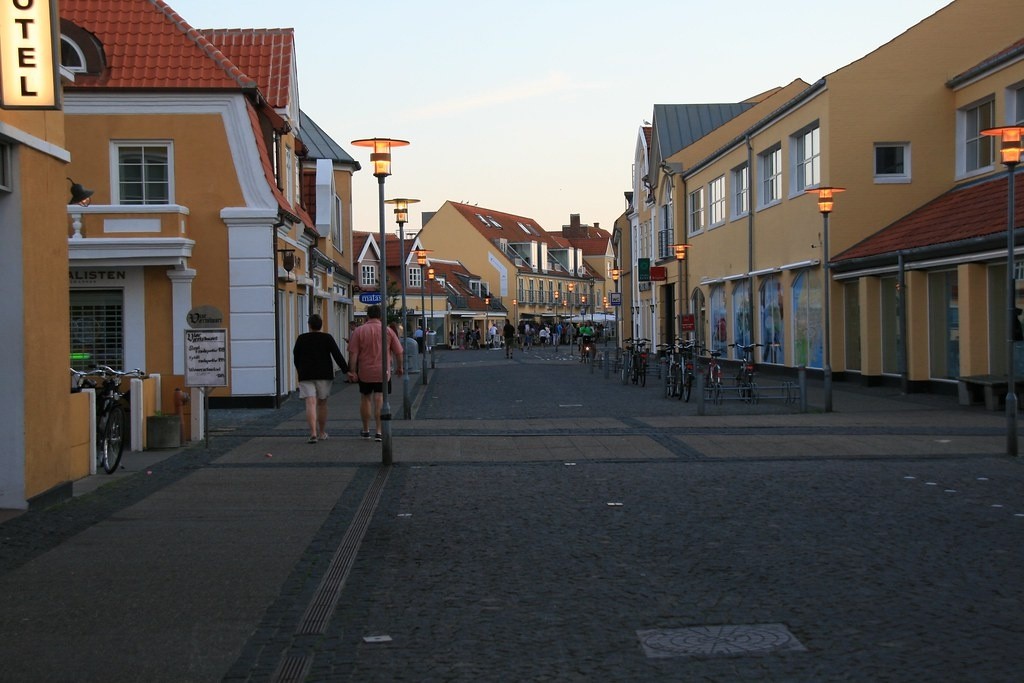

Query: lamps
<box><xmin>66</xmin><ymin>177</ymin><xmax>95</xmax><ymax>208</ymax></box>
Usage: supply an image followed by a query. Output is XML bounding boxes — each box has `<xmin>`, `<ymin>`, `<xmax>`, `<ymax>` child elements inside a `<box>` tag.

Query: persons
<box><xmin>293</xmin><ymin>314</ymin><xmax>355</xmax><ymax>445</ymax></box>
<box><xmin>347</xmin><ymin>306</ymin><xmax>404</xmax><ymax>442</ymax></box>
<box><xmin>344</xmin><ymin>319</ymin><xmax>359</xmax><ymax>385</ymax></box>
<box><xmin>465</xmin><ymin>318</ymin><xmax>609</xmax><ymax>364</ymax></box>
<box><xmin>414</xmin><ymin>325</ymin><xmax>424</xmax><ymax>354</ymax></box>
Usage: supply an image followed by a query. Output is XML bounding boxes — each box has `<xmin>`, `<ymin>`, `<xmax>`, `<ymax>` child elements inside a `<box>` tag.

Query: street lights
<box><xmin>603</xmin><ymin>296</ymin><xmax>607</xmax><ymax>347</ymax></box>
<box><xmin>563</xmin><ymin>301</ymin><xmax>566</xmax><ymax>326</ymax></box>
<box><xmin>606</xmin><ymin>266</ymin><xmax>621</xmax><ymax>346</ymax></box>
<box><xmin>428</xmin><ymin>267</ymin><xmax>435</xmax><ymax>368</ymax></box>
<box><xmin>382</xmin><ymin>198</ymin><xmax>420</xmax><ymax>420</ymax></box>
<box><xmin>804</xmin><ymin>187</ymin><xmax>847</xmax><ymax>413</ymax></box>
<box><xmin>411</xmin><ymin>247</ymin><xmax>435</xmax><ymax>385</ymax></box>
<box><xmin>569</xmin><ymin>282</ymin><xmax>574</xmax><ymax>354</ymax></box>
<box><xmin>582</xmin><ymin>297</ymin><xmax>586</xmax><ymax>325</ymax></box>
<box><xmin>351</xmin><ymin>136</ymin><xmax>411</xmax><ymax>466</ymax></box>
<box><xmin>512</xmin><ymin>299</ymin><xmax>517</xmax><ymax>332</ymax></box>
<box><xmin>485</xmin><ymin>297</ymin><xmax>489</xmax><ymax>349</ymax></box>
<box><xmin>555</xmin><ymin>291</ymin><xmax>558</xmax><ymax>352</ymax></box>
<box><xmin>981</xmin><ymin>125</ymin><xmax>1024</xmax><ymax>457</ymax></box>
<box><xmin>667</xmin><ymin>243</ymin><xmax>694</xmax><ymax>344</ymax></box>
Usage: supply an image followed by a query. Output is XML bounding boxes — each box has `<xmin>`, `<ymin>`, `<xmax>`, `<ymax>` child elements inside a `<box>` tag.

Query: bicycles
<box><xmin>620</xmin><ymin>337</ymin><xmax>765</xmax><ymax>403</ymax></box>
<box><xmin>69</xmin><ymin>364</ymin><xmax>144</xmax><ymax>474</ymax></box>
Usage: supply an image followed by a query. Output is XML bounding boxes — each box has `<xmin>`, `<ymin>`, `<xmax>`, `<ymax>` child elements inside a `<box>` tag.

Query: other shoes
<box><xmin>511</xmin><ymin>356</ymin><xmax>512</xmax><ymax>359</ymax></box>
<box><xmin>374</xmin><ymin>434</ymin><xmax>382</xmax><ymax>442</ymax></box>
<box><xmin>579</xmin><ymin>360</ymin><xmax>583</xmax><ymax>362</ymax></box>
<box><xmin>507</xmin><ymin>357</ymin><xmax>509</xmax><ymax>359</ymax></box>
<box><xmin>359</xmin><ymin>429</ymin><xmax>370</xmax><ymax>440</ymax></box>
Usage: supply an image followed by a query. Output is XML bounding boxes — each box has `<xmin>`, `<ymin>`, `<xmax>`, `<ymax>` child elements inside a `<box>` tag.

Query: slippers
<box><xmin>319</xmin><ymin>432</ymin><xmax>328</xmax><ymax>440</ymax></box>
<box><xmin>307</xmin><ymin>436</ymin><xmax>318</xmax><ymax>443</ymax></box>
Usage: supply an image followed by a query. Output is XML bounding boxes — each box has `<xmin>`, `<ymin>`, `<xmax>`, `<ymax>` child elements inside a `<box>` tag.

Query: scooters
<box><xmin>580</xmin><ymin>334</ymin><xmax>594</xmax><ymax>357</ymax></box>
